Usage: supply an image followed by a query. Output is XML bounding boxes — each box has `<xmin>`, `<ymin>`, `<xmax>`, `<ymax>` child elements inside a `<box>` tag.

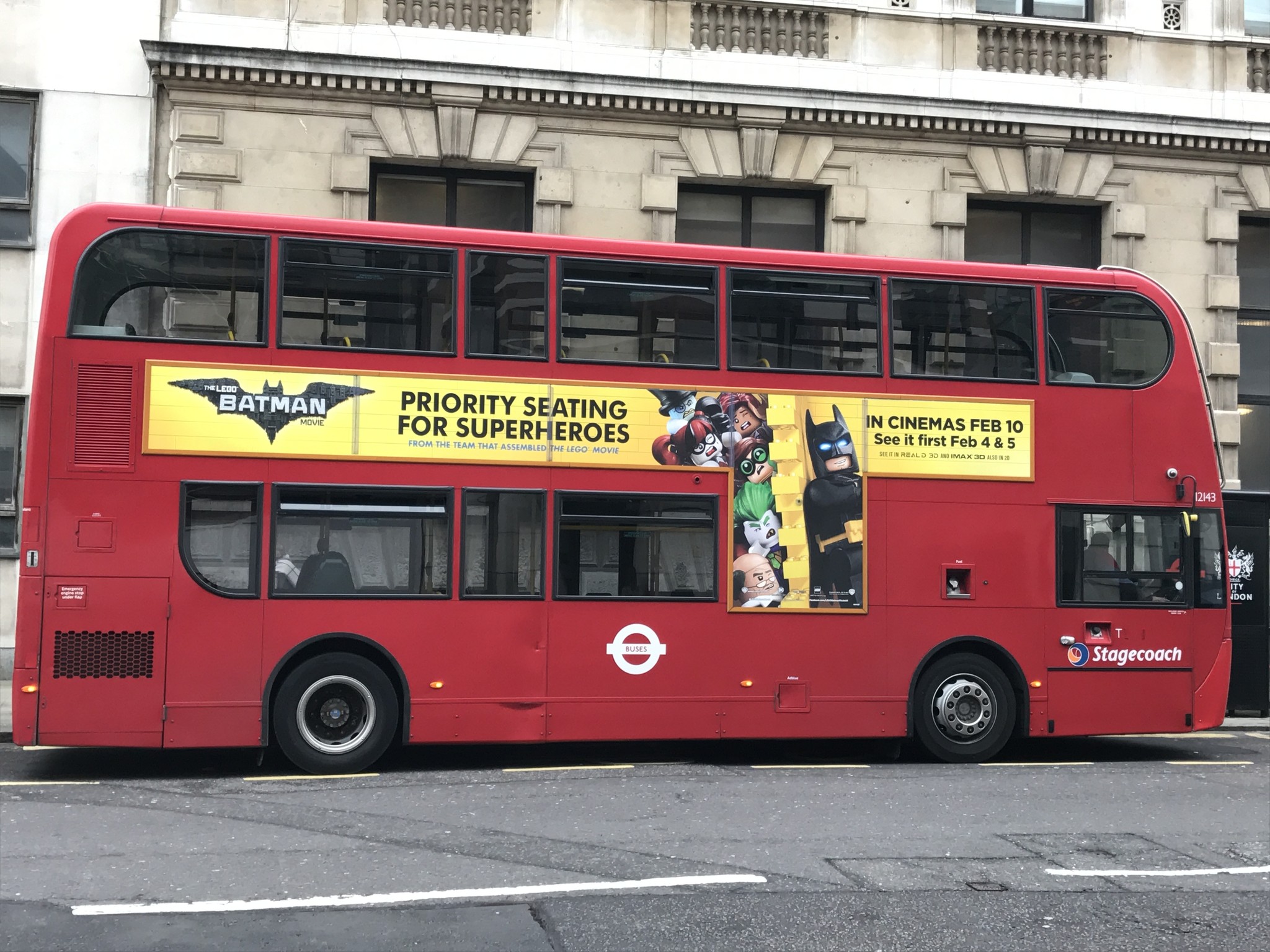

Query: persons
<box><xmin>1084</xmin><ymin>532</ymin><xmax>1120</xmax><ymax>571</ymax></box>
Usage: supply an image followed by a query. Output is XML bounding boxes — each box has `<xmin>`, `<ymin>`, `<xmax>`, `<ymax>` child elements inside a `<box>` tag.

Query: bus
<box><xmin>11</xmin><ymin>198</ymin><xmax>1234</xmax><ymax>766</ymax></box>
